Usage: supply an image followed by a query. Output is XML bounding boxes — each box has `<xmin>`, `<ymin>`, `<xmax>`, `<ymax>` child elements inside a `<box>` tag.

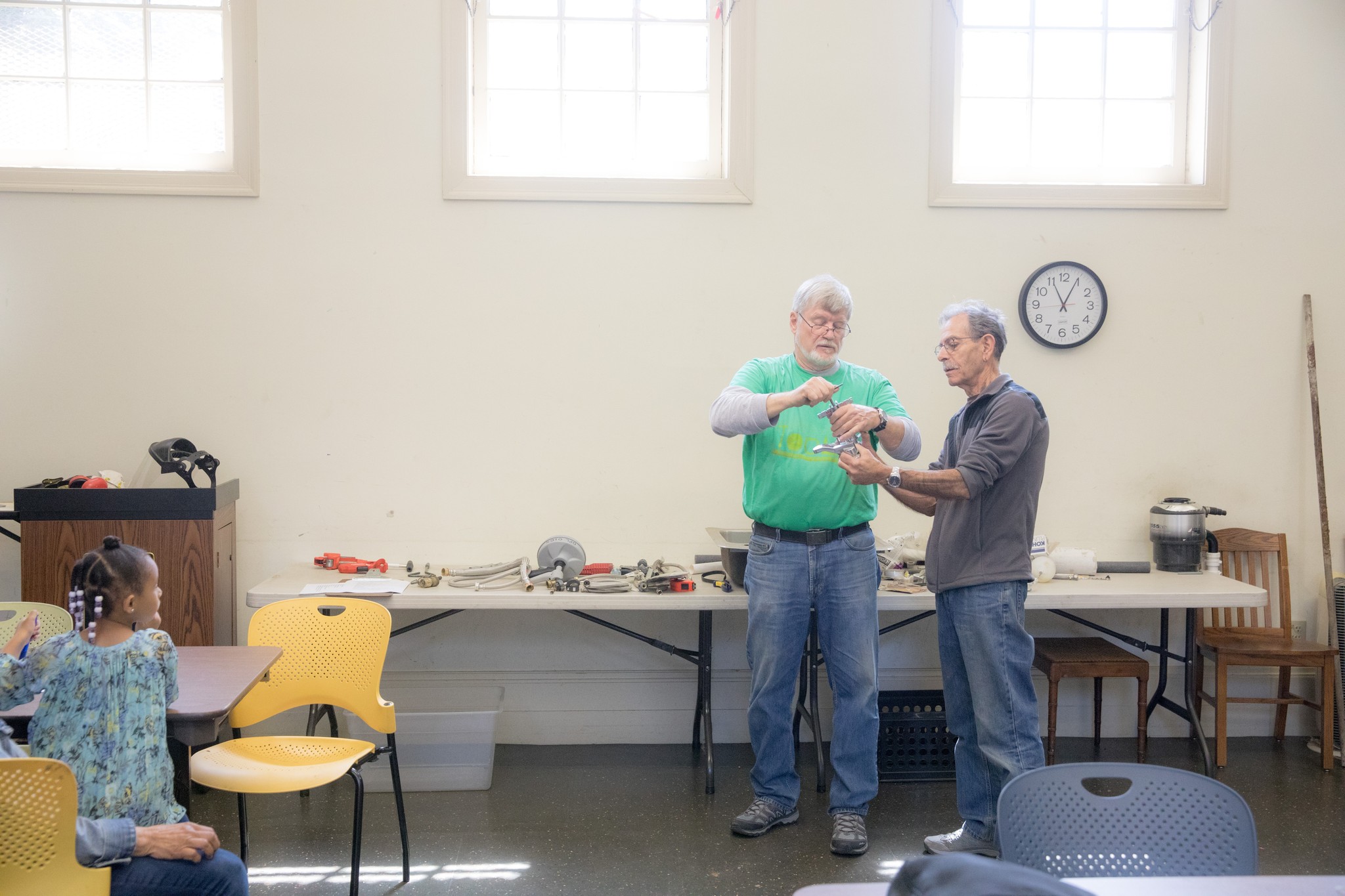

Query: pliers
<box><xmin>702</xmin><ymin>569</ymin><xmax>732</xmax><ymax>592</ymax></box>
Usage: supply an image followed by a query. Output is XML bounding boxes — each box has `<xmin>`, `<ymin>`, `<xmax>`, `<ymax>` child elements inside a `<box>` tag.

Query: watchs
<box><xmin>870</xmin><ymin>407</ymin><xmax>887</xmax><ymax>432</ymax></box>
<box><xmin>886</xmin><ymin>467</ymin><xmax>901</xmax><ymax>487</ymax></box>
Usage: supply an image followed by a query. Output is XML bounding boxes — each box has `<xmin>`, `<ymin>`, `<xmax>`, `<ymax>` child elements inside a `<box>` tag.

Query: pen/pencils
<box><xmin>17</xmin><ymin>615</ymin><xmax>38</xmax><ymax>662</ymax></box>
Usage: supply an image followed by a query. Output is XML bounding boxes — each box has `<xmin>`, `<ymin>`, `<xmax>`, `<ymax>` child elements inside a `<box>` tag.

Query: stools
<box><xmin>1026</xmin><ymin>634</ymin><xmax>1154</xmax><ymax>772</ymax></box>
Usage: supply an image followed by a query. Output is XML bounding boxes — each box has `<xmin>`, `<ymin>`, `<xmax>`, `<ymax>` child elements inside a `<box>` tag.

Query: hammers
<box><xmin>388</xmin><ymin>560</ymin><xmax>414</xmax><ymax>572</ymax></box>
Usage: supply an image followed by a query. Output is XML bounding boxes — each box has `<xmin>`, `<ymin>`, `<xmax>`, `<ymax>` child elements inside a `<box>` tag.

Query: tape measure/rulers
<box><xmin>670</xmin><ymin>580</ymin><xmax>696</xmax><ymax>591</ymax></box>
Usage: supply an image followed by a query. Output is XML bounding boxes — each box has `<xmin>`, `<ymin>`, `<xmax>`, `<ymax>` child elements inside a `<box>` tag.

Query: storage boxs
<box><xmin>341</xmin><ymin>683</ymin><xmax>507</xmax><ymax>793</ymax></box>
<box><xmin>873</xmin><ymin>687</ymin><xmax>956</xmax><ymax>783</ymax></box>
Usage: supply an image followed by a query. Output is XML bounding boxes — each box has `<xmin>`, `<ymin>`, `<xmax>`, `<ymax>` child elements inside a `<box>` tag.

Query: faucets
<box><xmin>811</xmin><ymin>382</ymin><xmax>862</xmax><ymax>460</ymax></box>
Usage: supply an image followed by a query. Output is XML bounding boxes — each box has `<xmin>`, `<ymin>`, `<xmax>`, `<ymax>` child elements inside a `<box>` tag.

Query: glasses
<box><xmin>935</xmin><ymin>335</ymin><xmax>983</xmax><ymax>356</ymax></box>
<box><xmin>796</xmin><ymin>311</ymin><xmax>851</xmax><ymax>338</ymax></box>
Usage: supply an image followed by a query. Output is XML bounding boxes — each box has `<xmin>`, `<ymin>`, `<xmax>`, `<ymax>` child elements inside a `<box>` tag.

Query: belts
<box><xmin>754</xmin><ymin>522</ymin><xmax>868</xmax><ymax>546</ymax></box>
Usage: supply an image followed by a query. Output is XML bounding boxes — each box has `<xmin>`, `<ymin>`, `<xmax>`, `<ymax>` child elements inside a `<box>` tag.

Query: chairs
<box><xmin>187</xmin><ymin>593</ymin><xmax>413</xmax><ymax>896</ymax></box>
<box><xmin>992</xmin><ymin>760</ymin><xmax>1262</xmax><ymax>878</ymax></box>
<box><xmin>1189</xmin><ymin>526</ymin><xmax>1339</xmax><ymax>774</ymax></box>
<box><xmin>0</xmin><ymin>755</ymin><xmax>113</xmax><ymax>896</ymax></box>
<box><xmin>0</xmin><ymin>599</ymin><xmax>75</xmax><ymax>653</ymax></box>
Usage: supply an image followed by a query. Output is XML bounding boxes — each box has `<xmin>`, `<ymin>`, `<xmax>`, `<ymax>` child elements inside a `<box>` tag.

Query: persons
<box><xmin>0</xmin><ymin>536</ymin><xmax>190</xmax><ymax>825</ymax></box>
<box><xmin>838</xmin><ymin>305</ymin><xmax>1050</xmax><ymax>862</ymax></box>
<box><xmin>712</xmin><ymin>273</ymin><xmax>921</xmax><ymax>857</ymax></box>
<box><xmin>0</xmin><ymin>719</ymin><xmax>249</xmax><ymax>896</ymax></box>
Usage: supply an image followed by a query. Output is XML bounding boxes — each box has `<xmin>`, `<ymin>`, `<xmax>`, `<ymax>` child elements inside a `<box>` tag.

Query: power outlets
<box><xmin>1290</xmin><ymin>619</ymin><xmax>1308</xmax><ymax>642</ymax></box>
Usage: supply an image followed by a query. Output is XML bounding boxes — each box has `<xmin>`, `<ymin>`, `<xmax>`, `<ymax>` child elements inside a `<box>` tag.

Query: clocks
<box><xmin>1018</xmin><ymin>256</ymin><xmax>1111</xmax><ymax>355</ymax></box>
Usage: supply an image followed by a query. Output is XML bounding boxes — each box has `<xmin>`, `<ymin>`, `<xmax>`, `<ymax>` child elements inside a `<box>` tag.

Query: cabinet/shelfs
<box><xmin>12</xmin><ymin>479</ymin><xmax>242</xmax><ymax>645</ymax></box>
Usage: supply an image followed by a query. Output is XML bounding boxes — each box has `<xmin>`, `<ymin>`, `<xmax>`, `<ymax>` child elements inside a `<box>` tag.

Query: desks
<box><xmin>789</xmin><ymin>870</ymin><xmax>1345</xmax><ymax>896</ymax></box>
<box><xmin>240</xmin><ymin>563</ymin><xmax>751</xmax><ymax>800</ymax></box>
<box><xmin>0</xmin><ymin>641</ymin><xmax>284</xmax><ymax>818</ymax></box>
<box><xmin>784</xmin><ymin>563</ymin><xmax>1270</xmax><ymax>795</ymax></box>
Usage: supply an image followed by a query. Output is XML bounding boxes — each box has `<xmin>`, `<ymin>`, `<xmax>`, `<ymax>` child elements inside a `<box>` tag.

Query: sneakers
<box><xmin>830</xmin><ymin>812</ymin><xmax>868</xmax><ymax>854</ymax></box>
<box><xmin>730</xmin><ymin>800</ymin><xmax>799</xmax><ymax>835</ymax></box>
<box><xmin>923</xmin><ymin>827</ymin><xmax>1000</xmax><ymax>857</ymax></box>
